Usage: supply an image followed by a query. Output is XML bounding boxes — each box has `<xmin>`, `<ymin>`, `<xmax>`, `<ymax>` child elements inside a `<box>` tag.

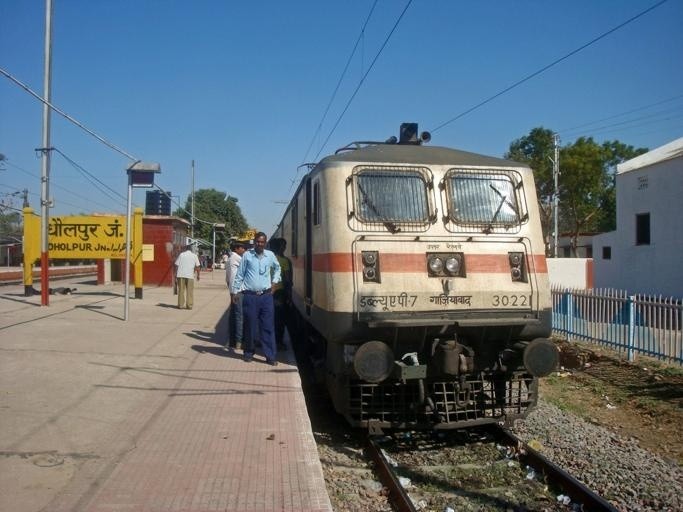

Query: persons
<box><xmin>220</xmin><ymin>252</ymin><xmax>228</xmax><ymax>269</ymax></box>
<box><xmin>173</xmin><ymin>244</ymin><xmax>201</xmax><ymax>309</ymax></box>
<box><xmin>224</xmin><ymin>230</ymin><xmax>293</xmax><ymax>367</ymax></box>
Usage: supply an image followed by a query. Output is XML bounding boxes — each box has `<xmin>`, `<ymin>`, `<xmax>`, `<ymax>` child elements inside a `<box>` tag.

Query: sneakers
<box><xmin>235</xmin><ymin>342</ymin><xmax>242</xmax><ymax>348</ymax></box>
<box><xmin>268</xmin><ymin>360</ymin><xmax>277</xmax><ymax>364</ymax></box>
<box><xmin>243</xmin><ymin>354</ymin><xmax>251</xmax><ymax>361</ymax></box>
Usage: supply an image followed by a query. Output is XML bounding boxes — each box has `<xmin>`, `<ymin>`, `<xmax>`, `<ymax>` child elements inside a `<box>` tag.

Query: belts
<box><xmin>244</xmin><ymin>290</ymin><xmax>271</xmax><ymax>295</ymax></box>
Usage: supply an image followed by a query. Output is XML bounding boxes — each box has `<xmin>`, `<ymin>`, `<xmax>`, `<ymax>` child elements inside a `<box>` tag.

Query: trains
<box><xmin>268</xmin><ymin>123</ymin><xmax>559</xmax><ymax>436</ymax></box>
